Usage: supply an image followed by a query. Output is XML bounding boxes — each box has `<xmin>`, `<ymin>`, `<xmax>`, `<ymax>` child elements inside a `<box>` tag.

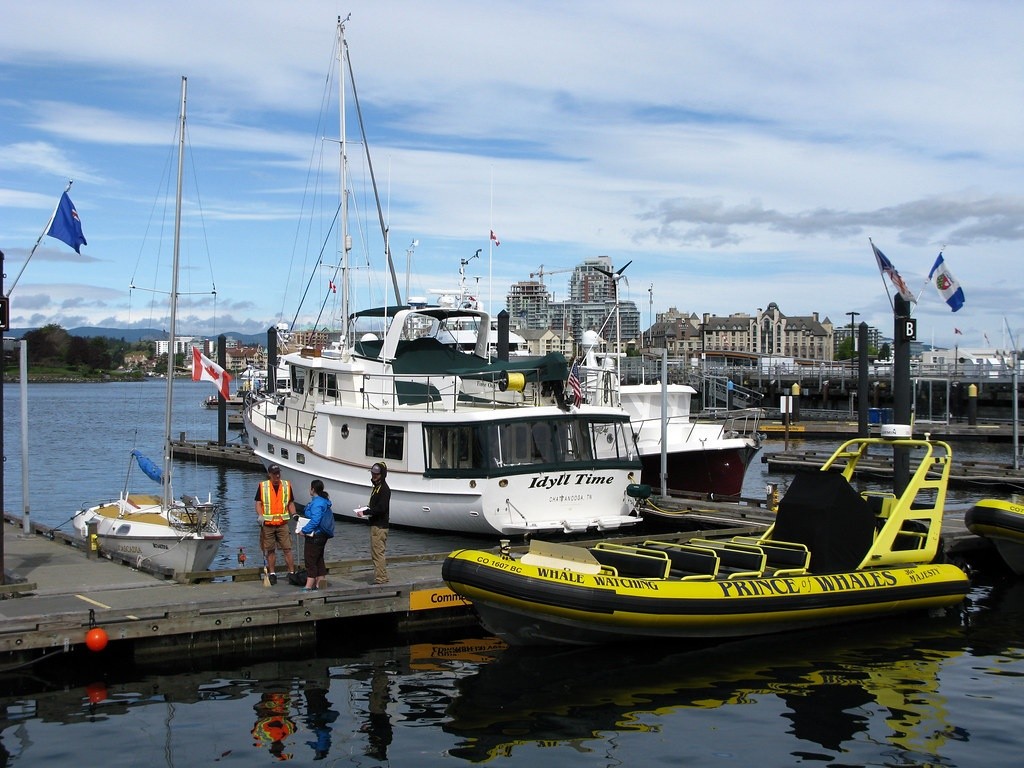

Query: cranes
<box><xmin>525</xmin><ymin>263</ymin><xmax>577</xmax><ymax>287</ymax></box>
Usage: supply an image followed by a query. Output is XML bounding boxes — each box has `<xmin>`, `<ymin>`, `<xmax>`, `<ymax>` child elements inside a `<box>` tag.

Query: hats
<box><xmin>268</xmin><ymin>464</ymin><xmax>281</xmax><ymax>472</ymax></box>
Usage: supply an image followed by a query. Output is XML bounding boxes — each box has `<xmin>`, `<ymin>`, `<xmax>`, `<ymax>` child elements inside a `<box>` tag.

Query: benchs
<box><xmin>728</xmin><ymin>537</ymin><xmax>812</xmax><ymax>577</ymax></box>
<box><xmin>685</xmin><ymin>538</ymin><xmax>767</xmax><ymax>581</ymax></box>
<box><xmin>642</xmin><ymin>541</ymin><xmax>720</xmax><ymax>581</ymax></box>
<box><xmin>590</xmin><ymin>543</ymin><xmax>671</xmax><ymax>581</ymax></box>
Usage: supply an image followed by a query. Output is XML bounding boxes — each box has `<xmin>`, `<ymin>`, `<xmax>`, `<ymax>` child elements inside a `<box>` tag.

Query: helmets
<box><xmin>369</xmin><ymin>462</ymin><xmax>388</xmax><ymax>475</ymax></box>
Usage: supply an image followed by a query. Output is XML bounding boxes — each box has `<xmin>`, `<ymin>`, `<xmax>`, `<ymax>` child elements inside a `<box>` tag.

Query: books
<box><xmin>353</xmin><ymin>505</ymin><xmax>371</xmax><ymax>517</ymax></box>
<box><xmin>295</xmin><ymin>517</ymin><xmax>315</xmax><ymax>537</ymax></box>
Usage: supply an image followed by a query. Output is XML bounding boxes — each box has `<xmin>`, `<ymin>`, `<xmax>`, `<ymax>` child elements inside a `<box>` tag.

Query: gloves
<box><xmin>292</xmin><ymin>513</ymin><xmax>301</xmax><ymax>522</ymax></box>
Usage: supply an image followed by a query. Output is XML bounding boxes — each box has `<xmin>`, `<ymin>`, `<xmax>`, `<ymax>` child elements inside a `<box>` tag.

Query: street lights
<box><xmin>845</xmin><ymin>311</ymin><xmax>861</xmax><ymax>379</ymax></box>
<box><xmin>4</xmin><ymin>338</ymin><xmax>35</xmax><ymax>538</ymax></box>
<box><xmin>698</xmin><ymin>323</ymin><xmax>710</xmax><ymax>361</ymax></box>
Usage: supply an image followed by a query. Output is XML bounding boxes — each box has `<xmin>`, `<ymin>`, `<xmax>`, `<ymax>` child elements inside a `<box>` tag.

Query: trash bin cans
<box><xmin>882</xmin><ymin>407</ymin><xmax>894</xmax><ymax>424</ymax></box>
<box><xmin>869</xmin><ymin>407</ymin><xmax>880</xmax><ymax>425</ymax></box>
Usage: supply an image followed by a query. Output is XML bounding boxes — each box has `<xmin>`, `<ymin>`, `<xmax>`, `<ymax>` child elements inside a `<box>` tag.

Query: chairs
<box><xmin>858</xmin><ymin>490</ymin><xmax>899</xmax><ymax>544</ymax></box>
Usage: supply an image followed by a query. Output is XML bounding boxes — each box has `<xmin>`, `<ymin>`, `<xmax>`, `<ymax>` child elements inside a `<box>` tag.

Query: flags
<box><xmin>568</xmin><ymin>361</ymin><xmax>582</xmax><ymax>410</ymax></box>
<box><xmin>45</xmin><ymin>190</ymin><xmax>88</xmax><ymax>256</ymax></box>
<box><xmin>191</xmin><ymin>345</ymin><xmax>231</xmax><ymax>401</ymax></box>
<box><xmin>490</xmin><ymin>230</ymin><xmax>500</xmax><ymax>246</ymax></box>
<box><xmin>329</xmin><ymin>282</ymin><xmax>336</xmax><ymax>292</ymax></box>
<box><xmin>929</xmin><ymin>252</ymin><xmax>965</xmax><ymax>312</ymax></box>
<box><xmin>871</xmin><ymin>243</ymin><xmax>918</xmax><ymax>306</ymax></box>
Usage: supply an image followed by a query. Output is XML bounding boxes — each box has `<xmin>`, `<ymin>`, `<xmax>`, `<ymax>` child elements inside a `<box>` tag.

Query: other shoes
<box><xmin>269</xmin><ymin>575</ymin><xmax>277</xmax><ymax>585</ymax></box>
<box><xmin>368</xmin><ymin>579</ymin><xmax>389</xmax><ymax>585</ymax></box>
<box><xmin>299</xmin><ymin>586</ymin><xmax>315</xmax><ymax>593</ymax></box>
<box><xmin>288</xmin><ymin>572</ymin><xmax>297</xmax><ymax>585</ymax></box>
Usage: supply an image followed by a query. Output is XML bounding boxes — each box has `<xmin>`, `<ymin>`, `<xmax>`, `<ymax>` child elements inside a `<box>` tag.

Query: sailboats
<box><xmin>234</xmin><ymin>12</ymin><xmax>648</xmax><ymax>542</ymax></box>
<box><xmin>70</xmin><ymin>75</ymin><xmax>225</xmax><ymax>585</ymax></box>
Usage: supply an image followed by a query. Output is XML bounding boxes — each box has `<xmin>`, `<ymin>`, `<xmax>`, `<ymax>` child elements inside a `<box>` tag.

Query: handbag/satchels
<box><xmin>294</xmin><ymin>517</ymin><xmax>315</xmax><ymax>538</ymax></box>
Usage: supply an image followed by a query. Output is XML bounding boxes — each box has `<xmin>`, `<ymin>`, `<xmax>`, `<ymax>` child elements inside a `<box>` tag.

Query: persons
<box><xmin>255</xmin><ymin>464</ymin><xmax>302</xmax><ymax>587</ymax></box>
<box><xmin>297</xmin><ymin>480</ymin><xmax>335</xmax><ymax>591</ymax></box>
<box><xmin>356</xmin><ymin>462</ymin><xmax>391</xmax><ymax>583</ymax></box>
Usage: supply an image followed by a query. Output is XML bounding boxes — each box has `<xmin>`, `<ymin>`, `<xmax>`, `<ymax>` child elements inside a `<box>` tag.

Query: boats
<box><xmin>574</xmin><ymin>328</ymin><xmax>767</xmax><ymax>504</ymax></box>
<box><xmin>438</xmin><ymin>422</ymin><xmax>973</xmax><ymax>660</ymax></box>
<box><xmin>964</xmin><ymin>478</ymin><xmax>1024</xmax><ymax>582</ymax></box>
